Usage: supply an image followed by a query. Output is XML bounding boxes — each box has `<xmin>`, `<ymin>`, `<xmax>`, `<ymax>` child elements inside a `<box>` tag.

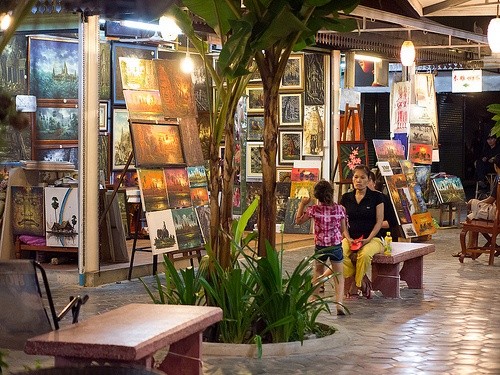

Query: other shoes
<box><xmin>306</xmin><ymin>279</ymin><xmax>373</xmax><ymax>316</ymax></box>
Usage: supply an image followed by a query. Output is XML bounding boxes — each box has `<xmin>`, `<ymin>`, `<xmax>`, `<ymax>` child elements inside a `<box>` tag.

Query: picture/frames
<box><xmin>245</xmin><ymin>52</ymin><xmax>326</xmax><ymax>184</ymax></box>
<box><xmin>31</xmin><ymin>143</ymin><xmax>79</xmax><ymax>171</ymax></box>
<box><xmin>128</xmin><ymin>119</ymin><xmax>188</xmax><ymax>169</ymax></box>
<box><xmin>25</xmin><ymin>34</ymin><xmax>79</xmax><ymax>104</ymax></box>
<box><xmin>110</xmin><ymin>44</ymin><xmax>158</xmax><ymax>103</ymax></box>
<box><xmin>30</xmin><ymin>103</ymin><xmax>79</xmax><ymax>144</ymax></box>
<box><xmin>97</xmin><ymin>98</ymin><xmax>112</xmax><ymax>134</ymax></box>
<box><xmin>337</xmin><ymin>141</ymin><xmax>369</xmax><ymax>182</ymax></box>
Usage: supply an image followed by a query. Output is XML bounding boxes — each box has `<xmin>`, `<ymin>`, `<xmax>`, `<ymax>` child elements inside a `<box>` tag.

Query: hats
<box><xmin>487</xmin><ymin>132</ymin><xmax>496</xmax><ymax>138</ymax></box>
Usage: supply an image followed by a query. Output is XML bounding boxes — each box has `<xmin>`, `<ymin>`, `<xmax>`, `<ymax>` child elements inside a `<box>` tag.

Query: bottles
<box><xmin>383</xmin><ymin>232</ymin><xmax>392</xmax><ymax>255</ymax></box>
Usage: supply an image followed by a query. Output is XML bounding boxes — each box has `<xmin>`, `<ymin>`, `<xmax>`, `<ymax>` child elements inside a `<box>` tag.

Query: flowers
<box><xmin>340</xmin><ymin>144</ymin><xmax>366</xmax><ymax>179</ymax></box>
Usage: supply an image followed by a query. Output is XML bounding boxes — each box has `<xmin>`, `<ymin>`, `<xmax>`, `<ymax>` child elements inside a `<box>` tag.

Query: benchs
<box><xmin>25</xmin><ymin>302</ymin><xmax>224</xmax><ymax>375</ymax></box>
<box><xmin>344</xmin><ymin>242</ymin><xmax>436</xmax><ymax>299</ymax></box>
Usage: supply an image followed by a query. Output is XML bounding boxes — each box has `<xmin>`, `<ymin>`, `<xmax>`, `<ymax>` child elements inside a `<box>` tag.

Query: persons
<box><xmin>452</xmin><ymin>155</ymin><xmax>500</xmax><ymax>259</ymax></box>
<box><xmin>295</xmin><ymin>180</ymin><xmax>349</xmax><ymax>316</ymax></box>
<box><xmin>366</xmin><ymin>171</ymin><xmax>397</xmax><ymax>241</ymax></box>
<box><xmin>479</xmin><ymin>133</ymin><xmax>500</xmax><ymax>199</ymax></box>
<box><xmin>337</xmin><ymin>166</ymin><xmax>385</xmax><ymax>300</ymax></box>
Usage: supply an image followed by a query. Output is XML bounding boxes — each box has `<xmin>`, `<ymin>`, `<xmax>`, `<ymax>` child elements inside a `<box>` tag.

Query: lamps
<box><xmin>180</xmin><ymin>36</ymin><xmax>195</xmax><ymax>73</ymax></box>
<box><xmin>159</xmin><ymin>15</ymin><xmax>179</xmax><ymax>40</ymax></box>
<box><xmin>486</xmin><ymin>17</ymin><xmax>500</xmax><ymax>54</ymax></box>
<box><xmin>399</xmin><ymin>40</ymin><xmax>415</xmax><ymax>67</ymax></box>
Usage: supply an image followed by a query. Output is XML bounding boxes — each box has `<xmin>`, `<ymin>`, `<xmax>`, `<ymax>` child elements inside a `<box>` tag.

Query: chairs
<box><xmin>458</xmin><ymin>182</ymin><xmax>500</xmax><ymax>266</ymax></box>
<box><xmin>0</xmin><ymin>259</ymin><xmax>90</xmax><ymax>349</ymax></box>
